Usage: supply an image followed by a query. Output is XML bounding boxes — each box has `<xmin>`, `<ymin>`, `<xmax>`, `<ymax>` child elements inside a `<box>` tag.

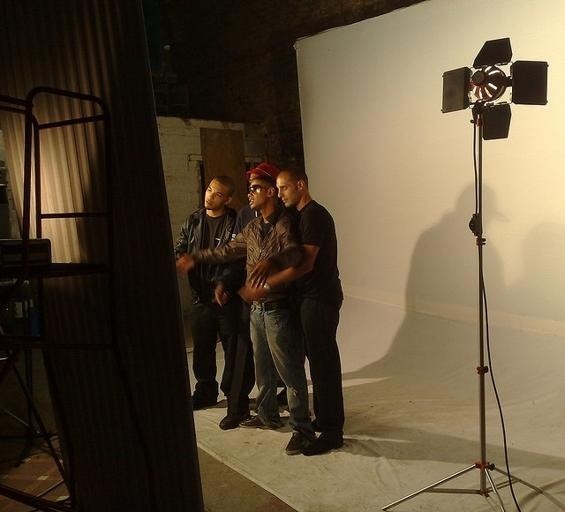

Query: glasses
<box><xmin>248</xmin><ymin>185</ymin><xmax>266</xmax><ymax>194</ymax></box>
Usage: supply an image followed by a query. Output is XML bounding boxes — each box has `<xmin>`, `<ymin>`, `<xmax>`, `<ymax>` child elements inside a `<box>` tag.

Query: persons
<box><xmin>218</xmin><ymin>162</ymin><xmax>288</xmax><ymax>430</ymax></box>
<box><xmin>175</xmin><ymin>174</ymin><xmax>317</xmax><ymax>456</ymax></box>
<box><xmin>237</xmin><ymin>168</ymin><xmax>346</xmax><ymax>457</ymax></box>
<box><xmin>174</xmin><ymin>175</ymin><xmax>237</xmax><ymax>409</ymax></box>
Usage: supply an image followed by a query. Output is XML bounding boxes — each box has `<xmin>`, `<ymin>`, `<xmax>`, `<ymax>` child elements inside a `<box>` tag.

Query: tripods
<box><xmin>381</xmin><ymin>112</ymin><xmax>544</xmax><ymax>512</ymax></box>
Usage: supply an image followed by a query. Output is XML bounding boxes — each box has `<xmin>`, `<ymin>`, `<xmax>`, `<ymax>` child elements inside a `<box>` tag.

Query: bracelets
<box><xmin>260</xmin><ymin>281</ymin><xmax>272</xmax><ymax>290</ymax></box>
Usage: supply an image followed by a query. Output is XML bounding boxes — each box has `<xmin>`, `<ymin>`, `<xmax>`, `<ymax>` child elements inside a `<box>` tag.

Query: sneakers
<box><xmin>192</xmin><ymin>388</ymin><xmax>344</xmax><ymax>456</ymax></box>
